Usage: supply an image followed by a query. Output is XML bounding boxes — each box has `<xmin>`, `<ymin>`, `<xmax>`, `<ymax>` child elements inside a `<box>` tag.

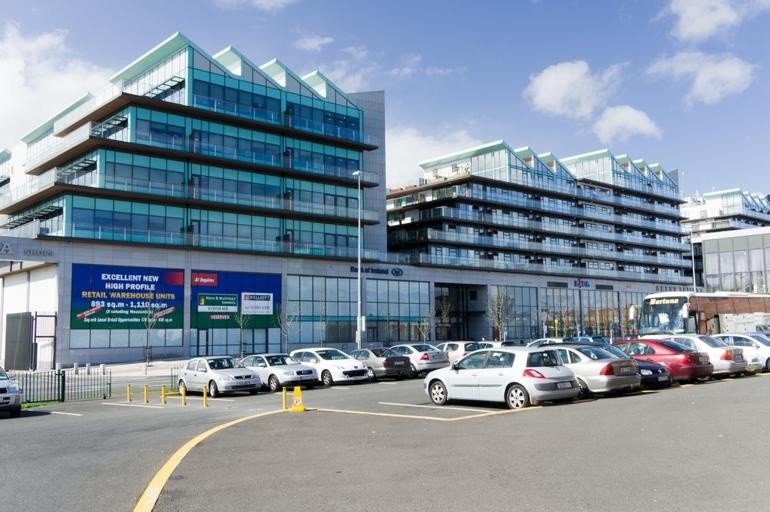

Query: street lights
<box><xmin>351</xmin><ymin>170</ymin><xmax>367</xmax><ymax>350</ymax></box>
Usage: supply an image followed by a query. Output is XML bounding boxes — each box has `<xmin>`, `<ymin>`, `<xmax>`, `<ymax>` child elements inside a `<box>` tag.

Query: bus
<box><xmin>629</xmin><ymin>290</ymin><xmax>770</xmax><ymax>339</ymax></box>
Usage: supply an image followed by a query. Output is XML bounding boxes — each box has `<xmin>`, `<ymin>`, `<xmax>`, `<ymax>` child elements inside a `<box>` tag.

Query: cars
<box><xmin>178</xmin><ymin>354</ymin><xmax>261</xmax><ymax>399</ymax></box>
<box><xmin>347</xmin><ymin>347</ymin><xmax>411</xmax><ymax>383</ymax></box>
<box><xmin>240</xmin><ymin>352</ymin><xmax>318</xmax><ymax>393</ymax></box>
<box><xmin>0</xmin><ymin>368</ymin><xmax>22</xmax><ymax>418</ymax></box>
<box><xmin>288</xmin><ymin>347</ymin><xmax>369</xmax><ymax>387</ymax></box>
<box><xmin>390</xmin><ymin>342</ymin><xmax>450</xmax><ymax>378</ymax></box>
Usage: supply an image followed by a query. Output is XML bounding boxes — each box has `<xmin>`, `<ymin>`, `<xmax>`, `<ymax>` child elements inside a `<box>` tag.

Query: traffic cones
<box><xmin>290</xmin><ymin>385</ymin><xmax>307</xmax><ymax>412</ymax></box>
